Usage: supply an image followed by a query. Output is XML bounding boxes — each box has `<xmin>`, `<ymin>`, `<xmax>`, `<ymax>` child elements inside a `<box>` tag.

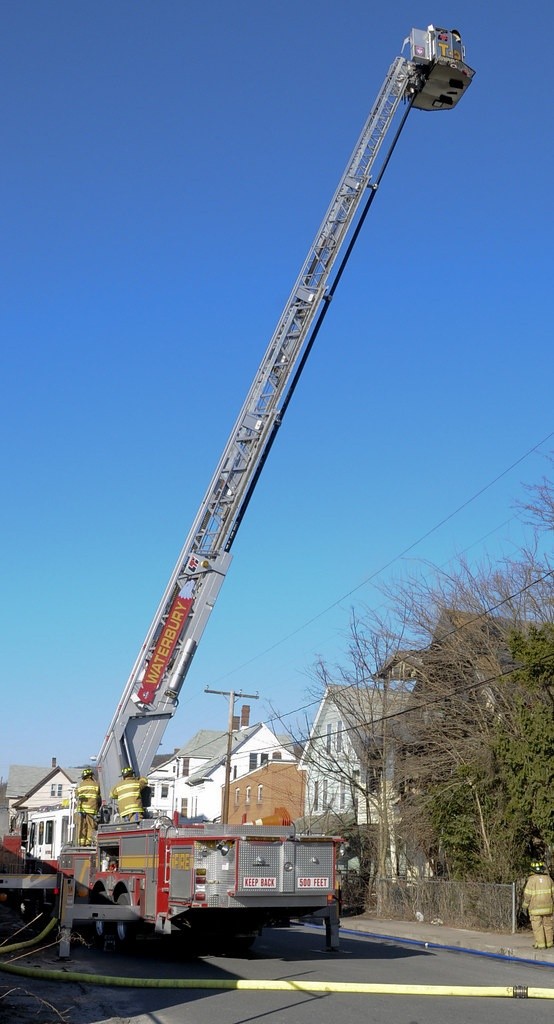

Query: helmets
<box><xmin>118</xmin><ymin>767</ymin><xmax>135</xmax><ymax>777</ymax></box>
<box><xmin>82</xmin><ymin>769</ymin><xmax>94</xmax><ymax>779</ymax></box>
<box><xmin>530</xmin><ymin>860</ymin><xmax>545</xmax><ymax>873</ymax></box>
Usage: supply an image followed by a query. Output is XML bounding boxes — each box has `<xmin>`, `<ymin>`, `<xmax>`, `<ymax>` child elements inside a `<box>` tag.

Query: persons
<box><xmin>521</xmin><ymin>859</ymin><xmax>554</xmax><ymax>950</ymax></box>
<box><xmin>74</xmin><ymin>769</ymin><xmax>101</xmax><ymax>847</ymax></box>
<box><xmin>109</xmin><ymin>767</ymin><xmax>149</xmax><ymax>822</ymax></box>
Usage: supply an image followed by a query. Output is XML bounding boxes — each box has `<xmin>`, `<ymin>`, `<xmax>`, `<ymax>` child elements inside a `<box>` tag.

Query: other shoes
<box><xmin>534</xmin><ymin>945</ymin><xmax>546</xmax><ymax>950</ymax></box>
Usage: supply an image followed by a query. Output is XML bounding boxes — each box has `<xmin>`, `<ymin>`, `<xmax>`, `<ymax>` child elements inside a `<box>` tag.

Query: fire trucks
<box><xmin>0</xmin><ymin>22</ymin><xmax>476</xmax><ymax>964</ymax></box>
